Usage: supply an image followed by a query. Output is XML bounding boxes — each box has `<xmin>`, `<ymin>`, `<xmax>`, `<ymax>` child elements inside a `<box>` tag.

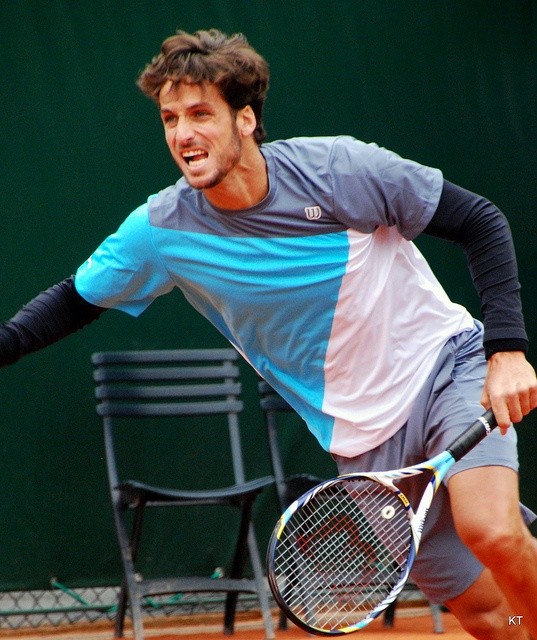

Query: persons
<box><xmin>0</xmin><ymin>25</ymin><xmax>537</xmax><ymax>640</ymax></box>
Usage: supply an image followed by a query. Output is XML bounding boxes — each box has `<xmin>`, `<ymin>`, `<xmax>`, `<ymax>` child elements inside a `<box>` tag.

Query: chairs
<box><xmin>92</xmin><ymin>349</ymin><xmax>277</xmax><ymax>640</ymax></box>
<box><xmin>257</xmin><ymin>375</ymin><xmax>444</xmax><ymax>634</ymax></box>
